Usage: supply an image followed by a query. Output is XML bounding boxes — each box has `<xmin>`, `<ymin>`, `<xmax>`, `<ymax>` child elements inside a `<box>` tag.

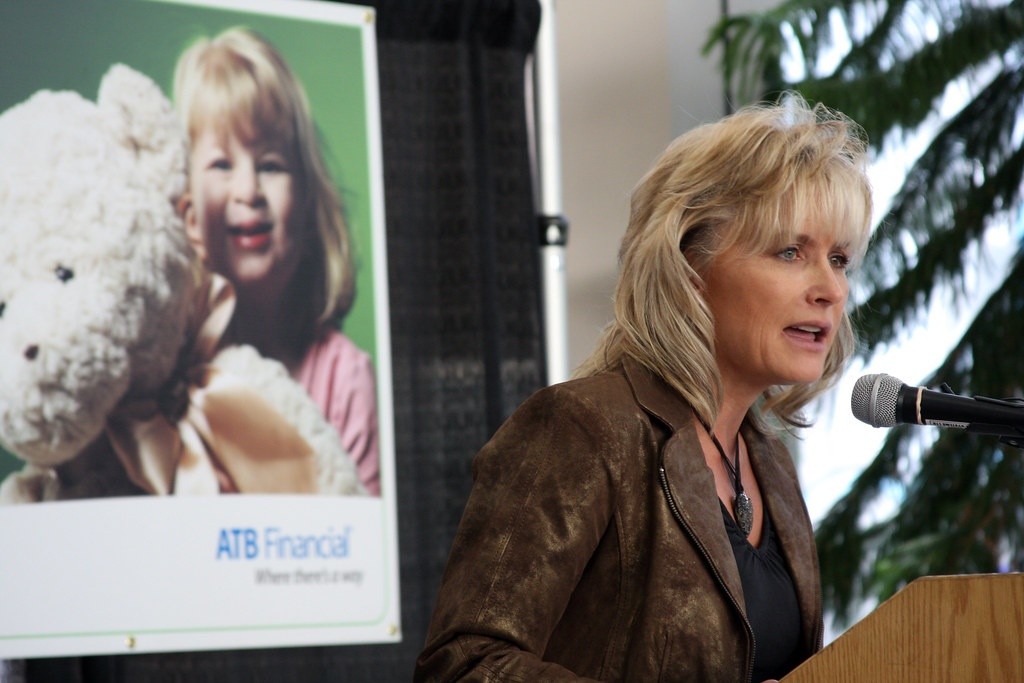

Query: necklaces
<box><xmin>693</xmin><ymin>407</ymin><xmax>754</xmax><ymax>541</ymax></box>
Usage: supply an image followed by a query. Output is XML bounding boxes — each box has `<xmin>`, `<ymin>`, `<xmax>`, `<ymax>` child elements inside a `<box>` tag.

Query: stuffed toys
<box><xmin>0</xmin><ymin>61</ymin><xmax>370</xmax><ymax>505</ymax></box>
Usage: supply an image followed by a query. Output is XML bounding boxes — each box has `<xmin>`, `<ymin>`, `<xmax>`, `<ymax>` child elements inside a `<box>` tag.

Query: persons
<box><xmin>410</xmin><ymin>89</ymin><xmax>874</xmax><ymax>683</ymax></box>
<box><xmin>1</xmin><ymin>27</ymin><xmax>384</xmax><ymax>504</ymax></box>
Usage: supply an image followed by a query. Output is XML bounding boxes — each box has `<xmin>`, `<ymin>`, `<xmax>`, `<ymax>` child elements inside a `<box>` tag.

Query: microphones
<box><xmin>851</xmin><ymin>373</ymin><xmax>1024</xmax><ymax>442</ymax></box>
